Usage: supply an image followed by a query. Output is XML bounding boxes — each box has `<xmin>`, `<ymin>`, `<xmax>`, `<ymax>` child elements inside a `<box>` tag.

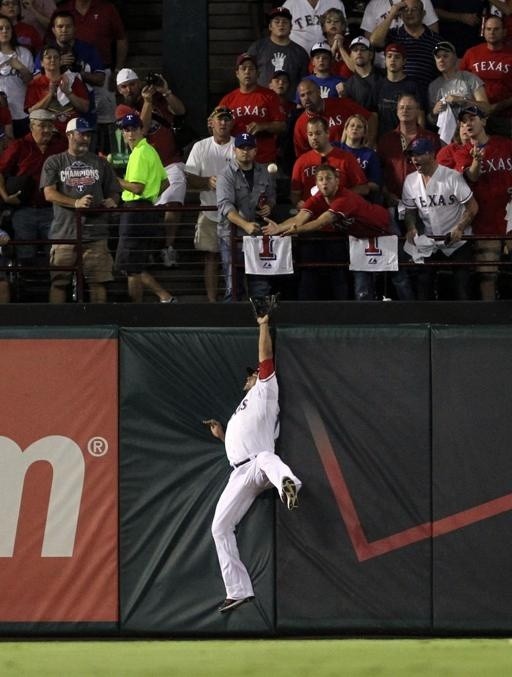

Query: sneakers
<box><xmin>17</xmin><ymin>261</ymin><xmax>40</xmax><ymax>283</ymax></box>
<box><xmin>218</xmin><ymin>596</ymin><xmax>253</xmax><ymax>614</ymax></box>
<box><xmin>280</xmin><ymin>476</ymin><xmax>297</xmax><ymax>510</ymax></box>
<box><xmin>159</xmin><ymin>245</ymin><xmax>179</xmax><ymax>269</ymax></box>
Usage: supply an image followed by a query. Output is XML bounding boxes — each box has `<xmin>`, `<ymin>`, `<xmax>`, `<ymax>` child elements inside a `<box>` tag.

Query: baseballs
<box><xmin>267</xmin><ymin>164</ymin><xmax>277</xmax><ymax>173</ymax></box>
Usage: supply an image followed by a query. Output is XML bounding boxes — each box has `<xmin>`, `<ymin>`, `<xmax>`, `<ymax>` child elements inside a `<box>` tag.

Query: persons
<box><xmin>202</xmin><ymin>296</ymin><xmax>302</xmax><ymax>612</ymax></box>
<box><xmin>0</xmin><ymin>0</ymin><xmax>512</xmax><ymax>301</ymax></box>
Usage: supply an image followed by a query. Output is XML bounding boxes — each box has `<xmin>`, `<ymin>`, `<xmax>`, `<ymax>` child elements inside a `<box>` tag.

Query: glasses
<box><xmin>215</xmin><ymin>108</ymin><xmax>234</xmax><ymax>115</ymax></box>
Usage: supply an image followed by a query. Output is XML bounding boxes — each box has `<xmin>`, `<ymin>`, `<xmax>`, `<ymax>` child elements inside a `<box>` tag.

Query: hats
<box><xmin>310</xmin><ymin>41</ymin><xmax>331</xmax><ymax>57</ymax></box>
<box><xmin>29</xmin><ymin>108</ymin><xmax>56</xmax><ymax>120</ymax></box>
<box><xmin>66</xmin><ymin>117</ymin><xmax>97</xmax><ymax>134</ymax></box>
<box><xmin>245</xmin><ymin>365</ymin><xmax>258</xmax><ymax>376</ymax></box>
<box><xmin>116</xmin><ymin>68</ymin><xmax>139</xmax><ymax>86</ymax></box>
<box><xmin>456</xmin><ymin>104</ymin><xmax>481</xmax><ymax>123</ymax></box>
<box><xmin>234</xmin><ymin>133</ymin><xmax>257</xmax><ymax>148</ymax></box>
<box><xmin>349</xmin><ymin>36</ymin><xmax>371</xmax><ymax>50</ymax></box>
<box><xmin>432</xmin><ymin>43</ymin><xmax>452</xmax><ymax>57</ymax></box>
<box><xmin>269</xmin><ymin>7</ymin><xmax>293</xmax><ymax>19</ymax></box>
<box><xmin>118</xmin><ymin>113</ymin><xmax>144</xmax><ymax>131</ymax></box>
<box><xmin>236</xmin><ymin>52</ymin><xmax>258</xmax><ymax>67</ymax></box>
<box><xmin>211</xmin><ymin>105</ymin><xmax>236</xmax><ymax>120</ymax></box>
<box><xmin>384</xmin><ymin>43</ymin><xmax>406</xmax><ymax>59</ymax></box>
<box><xmin>405</xmin><ymin>138</ymin><xmax>436</xmax><ymax>157</ymax></box>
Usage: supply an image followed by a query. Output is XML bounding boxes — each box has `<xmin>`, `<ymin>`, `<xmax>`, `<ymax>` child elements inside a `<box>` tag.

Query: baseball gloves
<box><xmin>250</xmin><ymin>295</ymin><xmax>281</xmax><ymax>328</ymax></box>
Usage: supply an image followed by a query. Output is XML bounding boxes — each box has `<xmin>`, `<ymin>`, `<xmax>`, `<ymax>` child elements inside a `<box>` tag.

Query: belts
<box><xmin>235</xmin><ymin>458</ymin><xmax>250</xmax><ymax>467</ymax></box>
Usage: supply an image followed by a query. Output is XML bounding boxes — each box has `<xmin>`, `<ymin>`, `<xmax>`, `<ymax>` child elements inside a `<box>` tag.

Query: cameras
<box><xmin>145</xmin><ymin>76</ymin><xmax>164</xmax><ymax>90</ymax></box>
